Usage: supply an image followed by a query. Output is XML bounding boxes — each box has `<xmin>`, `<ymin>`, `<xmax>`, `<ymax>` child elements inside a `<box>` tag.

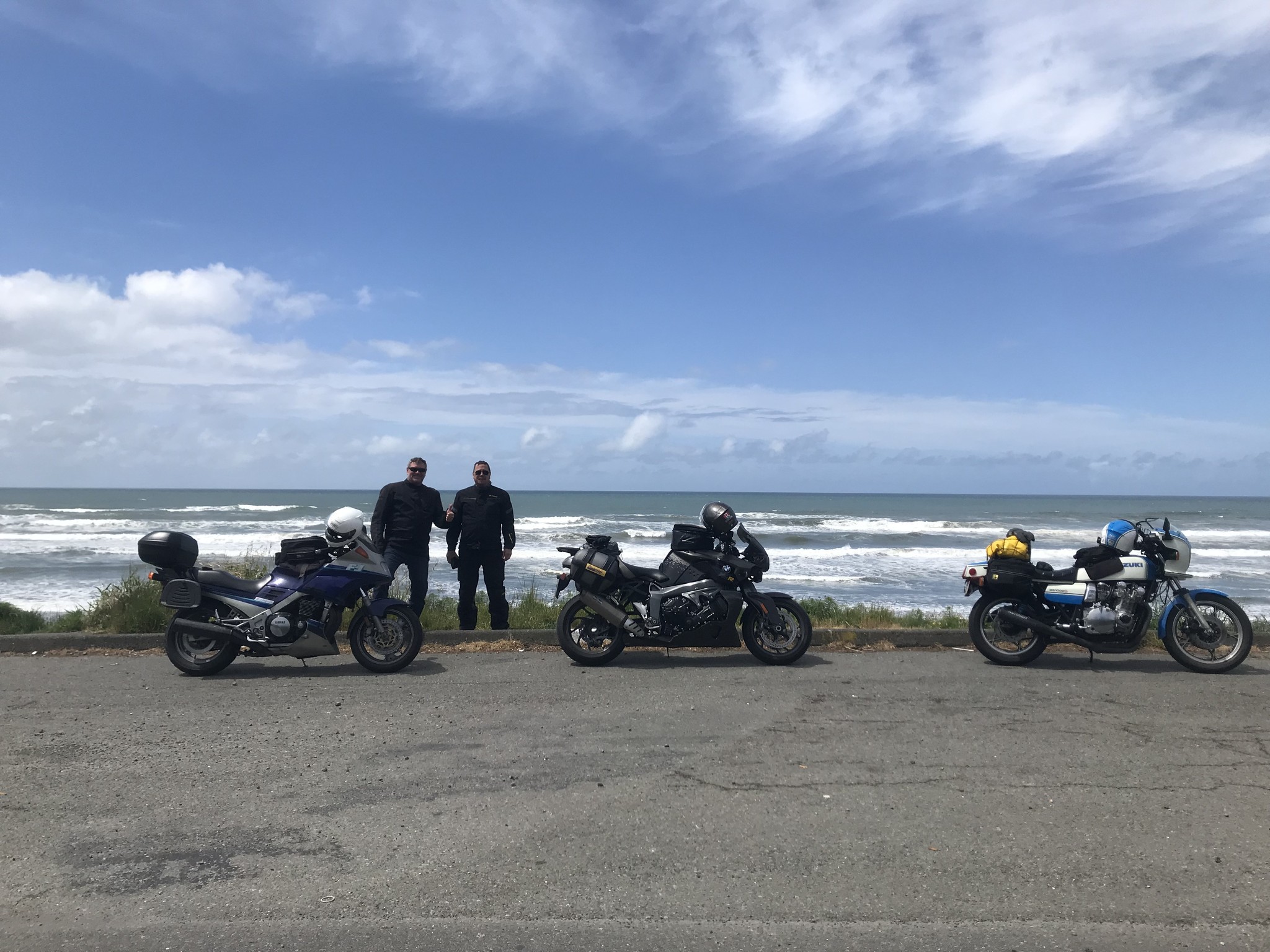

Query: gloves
<box><xmin>585</xmin><ymin>534</ymin><xmax>612</xmax><ymax>544</ymax></box>
<box><xmin>1035</xmin><ymin>561</ymin><xmax>1053</xmax><ymax>571</ymax></box>
<box><xmin>1007</xmin><ymin>528</ymin><xmax>1035</xmax><ymax>543</ymax></box>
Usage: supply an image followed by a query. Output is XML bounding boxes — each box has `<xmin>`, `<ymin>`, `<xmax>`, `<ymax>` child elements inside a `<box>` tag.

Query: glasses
<box><xmin>408</xmin><ymin>467</ymin><xmax>427</xmax><ymax>473</ymax></box>
<box><xmin>474</xmin><ymin>470</ymin><xmax>490</xmax><ymax>475</ymax></box>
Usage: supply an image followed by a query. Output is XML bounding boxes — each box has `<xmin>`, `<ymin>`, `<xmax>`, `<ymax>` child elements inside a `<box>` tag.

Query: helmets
<box><xmin>700</xmin><ymin>501</ymin><xmax>738</xmax><ymax>537</ymax></box>
<box><xmin>324</xmin><ymin>507</ymin><xmax>364</xmax><ymax>548</ymax></box>
<box><xmin>1097</xmin><ymin>519</ymin><xmax>1139</xmax><ymax>555</ymax></box>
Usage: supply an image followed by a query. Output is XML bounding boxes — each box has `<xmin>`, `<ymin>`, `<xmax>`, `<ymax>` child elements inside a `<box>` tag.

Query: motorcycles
<box><xmin>137</xmin><ymin>524</ymin><xmax>422</xmax><ymax>674</ymax></box>
<box><xmin>555</xmin><ymin>522</ymin><xmax>812</xmax><ymax>666</ymax></box>
<box><xmin>961</xmin><ymin>518</ymin><xmax>1254</xmax><ymax>674</ymax></box>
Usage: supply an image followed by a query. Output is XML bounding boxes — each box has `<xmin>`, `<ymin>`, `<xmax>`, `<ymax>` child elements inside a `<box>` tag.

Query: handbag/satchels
<box><xmin>275</xmin><ymin>536</ymin><xmax>332</xmax><ymax>579</ymax></box>
<box><xmin>1073</xmin><ymin>544</ymin><xmax>1123</xmax><ymax>581</ymax></box>
<box><xmin>671</xmin><ymin>523</ymin><xmax>714</xmax><ymax>551</ymax></box>
<box><xmin>986</xmin><ymin>535</ymin><xmax>1031</xmax><ymax>560</ymax></box>
<box><xmin>583</xmin><ymin>540</ymin><xmax>619</xmax><ymax>556</ymax></box>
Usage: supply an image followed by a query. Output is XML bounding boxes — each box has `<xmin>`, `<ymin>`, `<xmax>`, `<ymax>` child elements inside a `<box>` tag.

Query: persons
<box><xmin>370</xmin><ymin>456</ymin><xmax>454</xmax><ymax>620</ymax></box>
<box><xmin>446</xmin><ymin>460</ymin><xmax>516</xmax><ymax>630</ymax></box>
